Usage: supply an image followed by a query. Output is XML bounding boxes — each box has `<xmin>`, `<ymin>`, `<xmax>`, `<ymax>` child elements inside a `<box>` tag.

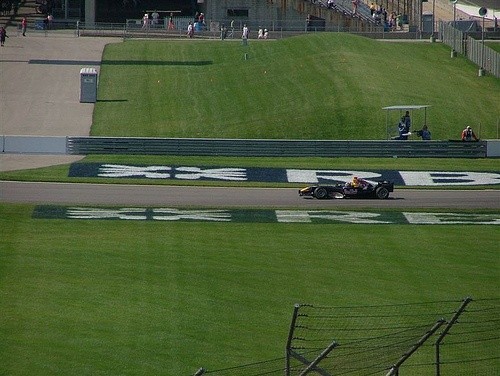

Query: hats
<box><xmin>467</xmin><ymin>126</ymin><xmax>471</xmax><ymax>129</ymax></box>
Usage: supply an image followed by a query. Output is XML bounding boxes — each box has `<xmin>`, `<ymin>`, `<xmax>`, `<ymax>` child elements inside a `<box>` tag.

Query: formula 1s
<box><xmin>298</xmin><ymin>179</ymin><xmax>394</xmax><ymax>200</ymax></box>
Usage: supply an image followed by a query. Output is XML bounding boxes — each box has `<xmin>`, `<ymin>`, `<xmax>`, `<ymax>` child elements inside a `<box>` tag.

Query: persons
<box><xmin>349</xmin><ymin>174</ymin><xmax>362</xmax><ymax>187</ymax></box>
<box><xmin>418</xmin><ymin>125</ymin><xmax>431</xmax><ymax>140</ymax></box>
<box><xmin>398</xmin><ymin>111</ymin><xmax>412</xmax><ymax>139</ymax></box>
<box><xmin>0</xmin><ymin>0</ymin><xmax>410</xmax><ymax>48</ymax></box>
<box><xmin>460</xmin><ymin>126</ymin><xmax>480</xmax><ymax>142</ymax></box>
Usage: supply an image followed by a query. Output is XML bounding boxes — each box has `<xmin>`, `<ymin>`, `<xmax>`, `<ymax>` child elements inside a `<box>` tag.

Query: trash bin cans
<box><xmin>79</xmin><ymin>67</ymin><xmax>98</xmax><ymax>103</ymax></box>
